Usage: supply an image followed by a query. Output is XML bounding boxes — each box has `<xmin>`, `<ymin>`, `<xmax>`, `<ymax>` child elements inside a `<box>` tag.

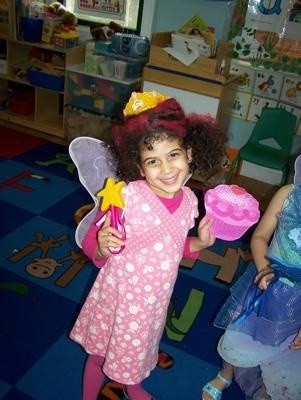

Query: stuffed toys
<box><xmin>91</xmin><ymin>20</ymin><xmax>124</xmax><ymax>41</ymax></box>
<box><xmin>49</xmin><ymin>1</ymin><xmax>78</xmax><ymax>25</ymax></box>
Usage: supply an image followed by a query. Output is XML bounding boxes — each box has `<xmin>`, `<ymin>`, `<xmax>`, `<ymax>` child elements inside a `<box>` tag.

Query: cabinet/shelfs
<box><xmin>1</xmin><ymin>0</ymin><xmax>86</xmax><ymax>140</ymax></box>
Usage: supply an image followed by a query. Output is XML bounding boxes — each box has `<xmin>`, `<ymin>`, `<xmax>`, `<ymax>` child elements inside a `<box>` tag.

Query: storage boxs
<box><xmin>66</xmin><ymin>49</ymin><xmax>149</xmax><ymax>119</ymax></box>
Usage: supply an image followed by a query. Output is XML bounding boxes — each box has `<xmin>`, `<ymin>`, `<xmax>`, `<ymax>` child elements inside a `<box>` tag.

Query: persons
<box><xmin>66</xmin><ymin>90</ymin><xmax>261</xmax><ymax>400</ymax></box>
<box><xmin>200</xmin><ymin>154</ymin><xmax>301</xmax><ymax>400</ymax></box>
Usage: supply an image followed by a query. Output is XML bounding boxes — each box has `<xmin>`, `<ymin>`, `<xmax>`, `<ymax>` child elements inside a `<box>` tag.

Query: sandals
<box><xmin>200</xmin><ymin>371</ymin><xmax>233</xmax><ymax>400</ymax></box>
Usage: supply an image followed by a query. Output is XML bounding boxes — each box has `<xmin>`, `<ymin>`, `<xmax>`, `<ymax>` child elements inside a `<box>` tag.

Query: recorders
<box><xmin>113</xmin><ymin>32</ymin><xmax>150</xmax><ymax>58</ymax></box>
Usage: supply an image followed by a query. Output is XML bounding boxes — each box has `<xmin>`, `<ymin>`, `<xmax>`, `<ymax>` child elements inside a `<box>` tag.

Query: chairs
<box><xmin>225</xmin><ymin>106</ymin><xmax>299</xmax><ymax>189</ymax></box>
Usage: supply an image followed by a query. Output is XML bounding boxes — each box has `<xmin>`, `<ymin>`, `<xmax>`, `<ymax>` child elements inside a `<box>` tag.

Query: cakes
<box><xmin>203</xmin><ymin>184</ymin><xmax>261</xmax><ymax>241</ymax></box>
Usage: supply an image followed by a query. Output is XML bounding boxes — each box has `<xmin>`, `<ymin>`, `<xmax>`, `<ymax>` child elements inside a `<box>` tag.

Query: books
<box><xmin>161</xmin><ymin>13</ymin><xmax>218</xmax><ymax>66</ymax></box>
